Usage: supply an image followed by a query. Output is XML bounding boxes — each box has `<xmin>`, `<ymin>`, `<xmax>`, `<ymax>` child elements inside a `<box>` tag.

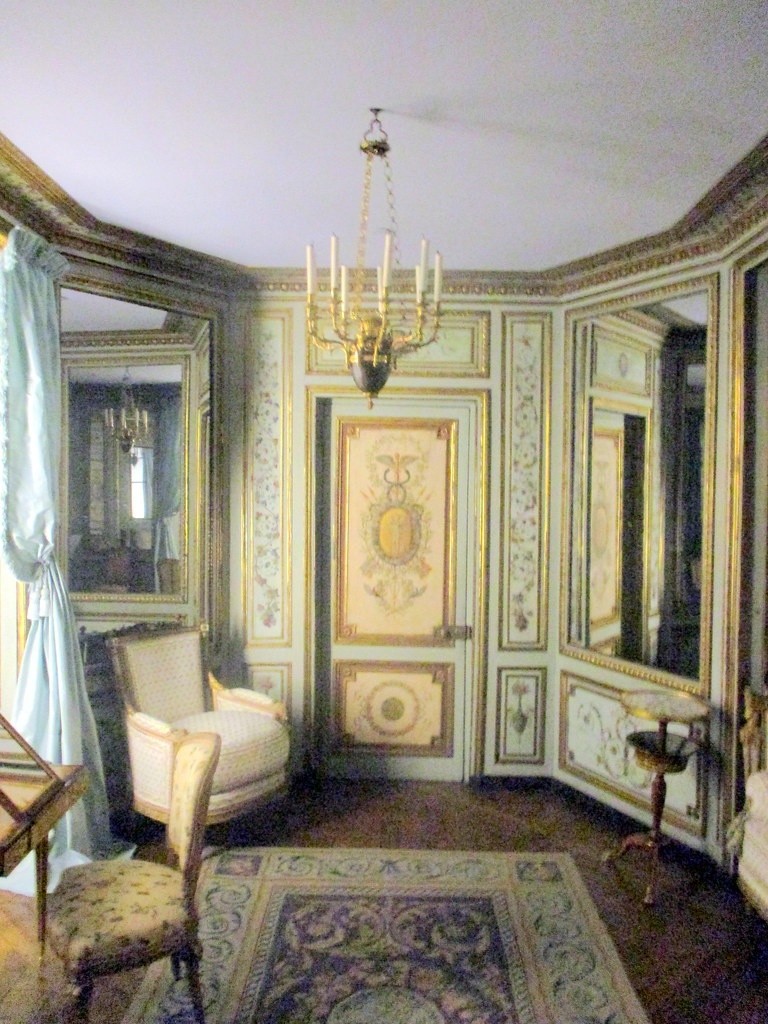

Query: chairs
<box><xmin>47</xmin><ymin>732</ymin><xmax>222</xmax><ymax>1024</ymax></box>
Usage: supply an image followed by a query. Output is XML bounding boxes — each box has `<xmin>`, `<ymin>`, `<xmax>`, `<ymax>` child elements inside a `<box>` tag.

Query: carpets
<box><xmin>120</xmin><ymin>845</ymin><xmax>654</xmax><ymax>1024</ymax></box>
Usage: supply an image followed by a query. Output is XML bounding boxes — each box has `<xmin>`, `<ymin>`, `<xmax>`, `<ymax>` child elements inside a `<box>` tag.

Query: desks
<box><xmin>600</xmin><ymin>689</ymin><xmax>711</xmax><ymax>908</ymax></box>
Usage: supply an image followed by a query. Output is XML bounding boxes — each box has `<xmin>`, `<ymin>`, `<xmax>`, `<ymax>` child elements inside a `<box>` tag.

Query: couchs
<box><xmin>109</xmin><ymin>623</ymin><xmax>291</xmax><ymax>827</ymax></box>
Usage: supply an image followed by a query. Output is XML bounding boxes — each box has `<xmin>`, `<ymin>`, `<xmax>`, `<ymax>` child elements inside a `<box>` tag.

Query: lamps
<box><xmin>306</xmin><ymin>108</ymin><xmax>442</xmax><ymax>408</ymax></box>
<box><xmin>103</xmin><ymin>365</ymin><xmax>148</xmax><ymax>451</ymax></box>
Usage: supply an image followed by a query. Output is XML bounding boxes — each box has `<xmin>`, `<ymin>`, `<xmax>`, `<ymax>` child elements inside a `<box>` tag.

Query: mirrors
<box><xmin>53</xmin><ymin>271</ymin><xmax>223</xmax><ymax>622</ymax></box>
<box><xmin>558</xmin><ymin>270</ymin><xmax>719</xmax><ymax>700</ymax></box>
